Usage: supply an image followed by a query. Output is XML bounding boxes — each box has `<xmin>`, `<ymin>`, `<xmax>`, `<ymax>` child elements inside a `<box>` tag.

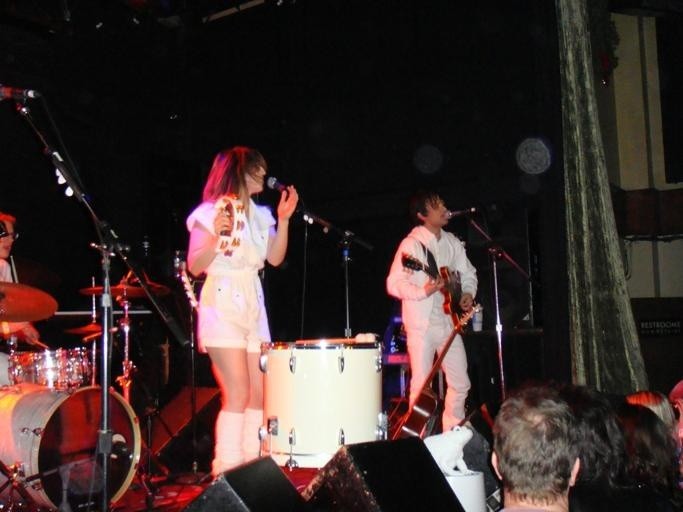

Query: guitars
<box><xmin>392</xmin><ymin>304</ymin><xmax>484</xmax><ymax>440</ymax></box>
<box><xmin>401</xmin><ymin>253</ymin><xmax>468</xmax><ymax>334</ymax></box>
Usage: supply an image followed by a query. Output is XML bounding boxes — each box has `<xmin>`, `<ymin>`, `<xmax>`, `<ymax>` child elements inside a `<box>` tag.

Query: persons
<box><xmin>384</xmin><ymin>186</ymin><xmax>480</xmax><ymax>435</ymax></box>
<box><xmin>0</xmin><ymin>213</ymin><xmax>50</xmax><ymax>351</ymax></box>
<box><xmin>490</xmin><ymin>377</ymin><xmax>683</xmax><ymax>512</ymax></box>
<box><xmin>187</xmin><ymin>146</ymin><xmax>300</xmax><ymax>482</ymax></box>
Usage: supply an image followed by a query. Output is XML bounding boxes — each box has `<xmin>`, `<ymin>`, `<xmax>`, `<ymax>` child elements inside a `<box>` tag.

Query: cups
<box><xmin>471</xmin><ymin>307</ymin><xmax>483</xmax><ymax>331</ymax></box>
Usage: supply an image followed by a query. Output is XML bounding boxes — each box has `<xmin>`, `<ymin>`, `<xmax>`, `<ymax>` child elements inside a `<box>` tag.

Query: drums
<box><xmin>259</xmin><ymin>340</ymin><xmax>389</xmax><ymax>469</ymax></box>
<box><xmin>0</xmin><ymin>383</ymin><xmax>142</xmax><ymax>512</ymax></box>
<box><xmin>8</xmin><ymin>347</ymin><xmax>91</xmax><ymax>386</ymax></box>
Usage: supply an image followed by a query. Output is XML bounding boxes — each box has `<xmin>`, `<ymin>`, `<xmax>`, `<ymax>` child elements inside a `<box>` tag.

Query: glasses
<box><xmin>425</xmin><ymin>200</ymin><xmax>446</xmax><ymax>211</ymax></box>
<box><xmin>0</xmin><ymin>231</ymin><xmax>20</xmax><ymax>243</ymax></box>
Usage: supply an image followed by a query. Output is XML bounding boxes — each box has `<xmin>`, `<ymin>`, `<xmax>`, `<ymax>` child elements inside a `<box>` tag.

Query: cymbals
<box><xmin>66</xmin><ymin>323</ymin><xmax>115</xmax><ymax>334</ymax></box>
<box><xmin>0</xmin><ymin>282</ymin><xmax>59</xmax><ymax>321</ymax></box>
<box><xmin>78</xmin><ymin>285</ymin><xmax>171</xmax><ymax>298</ymax></box>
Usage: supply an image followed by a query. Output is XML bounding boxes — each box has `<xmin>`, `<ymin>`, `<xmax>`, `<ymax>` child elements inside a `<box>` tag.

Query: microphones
<box><xmin>267</xmin><ymin>176</ymin><xmax>291</xmax><ymax>196</ymax></box>
<box><xmin>0</xmin><ymin>83</ymin><xmax>38</xmax><ymax>100</ymax></box>
<box><xmin>443</xmin><ymin>206</ymin><xmax>477</xmax><ymax>220</ymax></box>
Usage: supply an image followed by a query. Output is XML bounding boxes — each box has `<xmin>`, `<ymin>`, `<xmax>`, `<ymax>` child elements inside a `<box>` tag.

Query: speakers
<box><xmin>178</xmin><ymin>456</ymin><xmax>313</xmax><ymax>512</ymax></box>
<box><xmin>302</xmin><ymin>437</ymin><xmax>465</xmax><ymax>512</ymax></box>
